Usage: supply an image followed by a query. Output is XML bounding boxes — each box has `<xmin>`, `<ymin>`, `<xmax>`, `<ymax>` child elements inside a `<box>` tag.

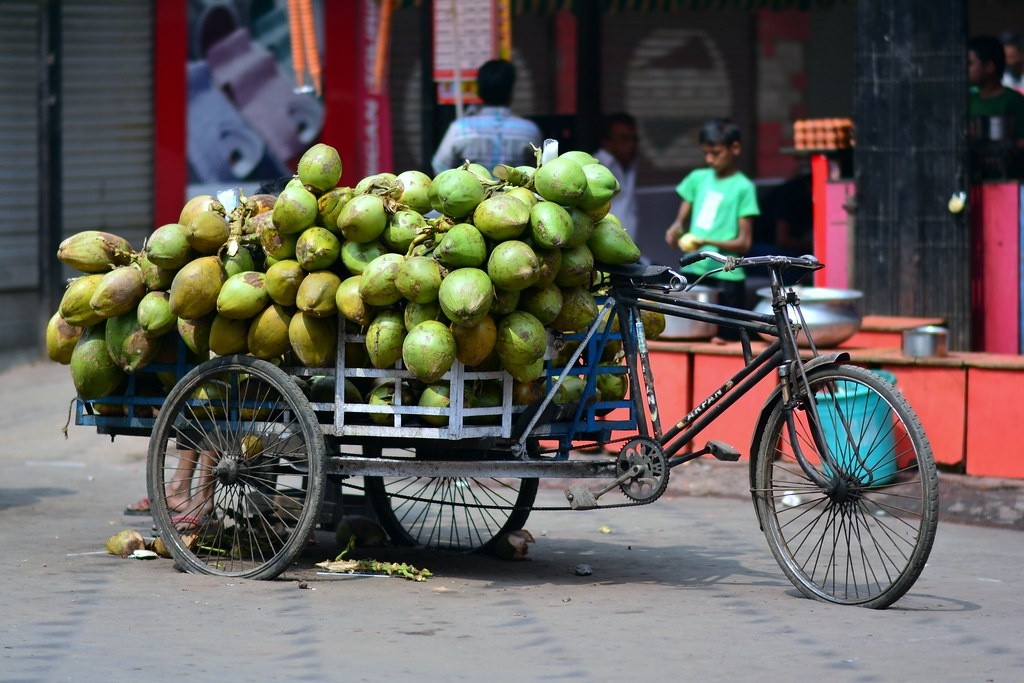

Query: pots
<box><xmin>642</xmin><ymin>284</ymin><xmax>720</xmax><ymax>339</ymax></box>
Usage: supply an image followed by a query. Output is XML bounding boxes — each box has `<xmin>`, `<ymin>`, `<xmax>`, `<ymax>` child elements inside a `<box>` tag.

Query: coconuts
<box><xmin>46</xmin><ymin>143</ymin><xmax>666</xmax><ymax>426</ymax></box>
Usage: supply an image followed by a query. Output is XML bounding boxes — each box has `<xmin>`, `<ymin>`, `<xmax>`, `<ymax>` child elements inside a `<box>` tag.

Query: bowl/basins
<box><xmin>752</xmin><ymin>287</ymin><xmax>863</xmax><ymax>348</ymax></box>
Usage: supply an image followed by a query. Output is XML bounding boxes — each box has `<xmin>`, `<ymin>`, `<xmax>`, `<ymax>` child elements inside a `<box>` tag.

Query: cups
<box><xmin>902</xmin><ymin>327</ymin><xmax>949</xmax><ymax>358</ymax></box>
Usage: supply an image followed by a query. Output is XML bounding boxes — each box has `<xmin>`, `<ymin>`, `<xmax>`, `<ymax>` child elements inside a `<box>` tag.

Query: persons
<box><xmin>125</xmin><ymin>448</ymin><xmax>227</xmax><ymax>533</ymax></box>
<box><xmin>746</xmin><ymin>148</ymin><xmax>853</xmax><ymax>286</ymax></box>
<box><xmin>665</xmin><ymin>116</ymin><xmax>761</xmax><ymax>341</ymax></box>
<box><xmin>430</xmin><ymin>58</ymin><xmax>541</xmax><ymax>182</ymax></box>
<box><xmin>967</xmin><ymin>35</ymin><xmax>1024</xmax><ymax>188</ymax></box>
<box><xmin>591</xmin><ymin>111</ymin><xmax>652</xmax><ymax>265</ymax></box>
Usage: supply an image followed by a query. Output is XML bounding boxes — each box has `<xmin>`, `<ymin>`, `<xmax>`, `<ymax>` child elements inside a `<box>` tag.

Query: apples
<box><xmin>678</xmin><ymin>234</ymin><xmax>698</xmax><ymax>252</ymax></box>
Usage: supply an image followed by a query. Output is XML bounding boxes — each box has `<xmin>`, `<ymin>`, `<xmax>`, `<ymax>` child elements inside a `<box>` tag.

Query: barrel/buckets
<box><xmin>816</xmin><ymin>370</ymin><xmax>896</xmax><ymax>492</ymax></box>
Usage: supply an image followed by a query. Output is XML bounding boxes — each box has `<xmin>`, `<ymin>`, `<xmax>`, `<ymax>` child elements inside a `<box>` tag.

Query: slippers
<box><xmin>123</xmin><ymin>495</ymin><xmax>181</xmax><ymax>517</ymax></box>
<box><xmin>150</xmin><ymin>514</ymin><xmax>214</xmax><ymax>537</ymax></box>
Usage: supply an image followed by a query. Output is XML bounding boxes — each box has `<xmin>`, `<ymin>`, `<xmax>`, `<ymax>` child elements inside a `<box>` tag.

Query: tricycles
<box><xmin>68</xmin><ymin>249</ymin><xmax>941</xmax><ymax>610</ymax></box>
